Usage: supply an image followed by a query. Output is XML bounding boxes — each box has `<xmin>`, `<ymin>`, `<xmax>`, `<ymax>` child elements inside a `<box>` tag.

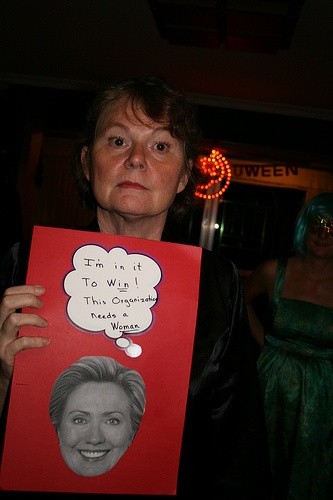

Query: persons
<box><xmin>243</xmin><ymin>194</ymin><xmax>333</xmax><ymax>500</ymax></box>
<box><xmin>0</xmin><ymin>72</ymin><xmax>273</xmax><ymax>500</ymax></box>
<box><xmin>50</xmin><ymin>355</ymin><xmax>147</xmax><ymax>478</ymax></box>
